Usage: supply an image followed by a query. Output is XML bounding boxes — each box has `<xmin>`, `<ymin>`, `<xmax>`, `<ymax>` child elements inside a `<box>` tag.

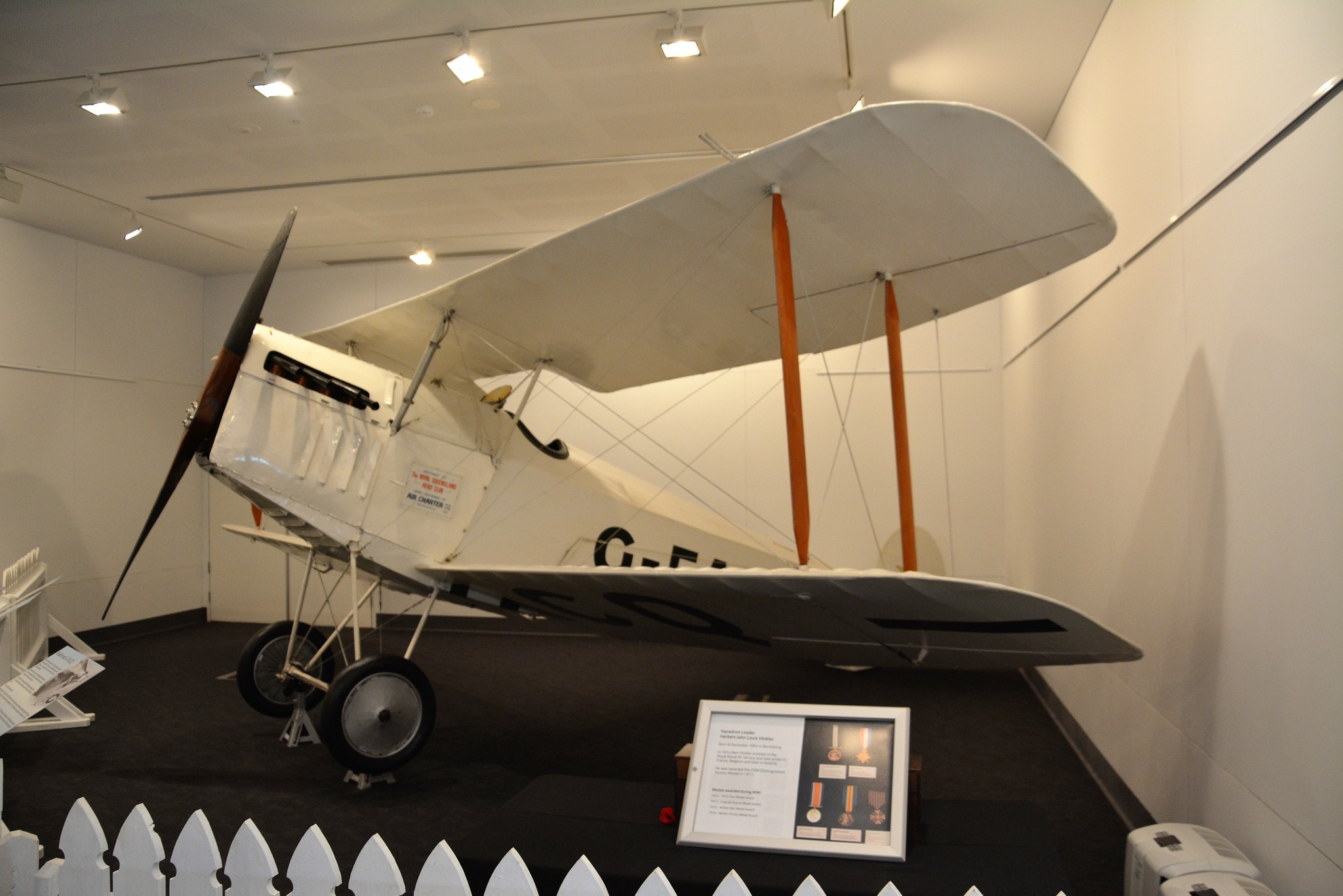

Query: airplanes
<box><xmin>98</xmin><ymin>100</ymin><xmax>1146</xmax><ymax>776</ymax></box>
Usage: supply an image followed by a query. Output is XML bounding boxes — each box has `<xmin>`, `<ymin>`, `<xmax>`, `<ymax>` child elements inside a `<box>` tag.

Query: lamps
<box><xmin>441</xmin><ymin>29</ymin><xmax>492</xmax><ymax>86</ymax></box>
<box><xmin>71</xmin><ymin>68</ymin><xmax>131</xmax><ymax>118</ymax></box>
<box><xmin>650</xmin><ymin>8</ymin><xmax>711</xmax><ymax>63</ymax></box>
<box><xmin>838</xmin><ymin>78</ymin><xmax>866</xmax><ymax>115</ymax></box>
<box><xmin>246</xmin><ymin>50</ymin><xmax>300</xmax><ymax>101</ymax></box>
<box><xmin>822</xmin><ymin>0</ymin><xmax>851</xmax><ymax>18</ymax></box>
